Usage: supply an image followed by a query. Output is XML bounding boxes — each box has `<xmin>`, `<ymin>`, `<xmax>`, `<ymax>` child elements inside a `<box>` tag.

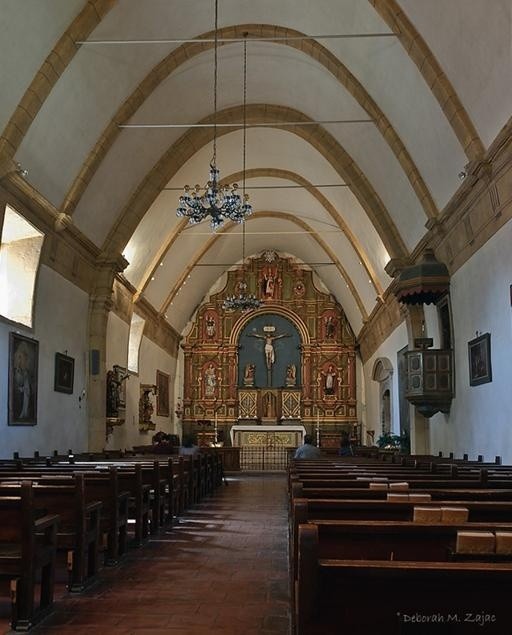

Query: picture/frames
<box><xmin>54</xmin><ymin>351</ymin><xmax>75</xmax><ymax>394</ymax></box>
<box><xmin>156</xmin><ymin>369</ymin><xmax>169</xmax><ymax>417</ymax></box>
<box><xmin>8</xmin><ymin>331</ymin><xmax>39</xmax><ymax>426</ymax></box>
<box><xmin>113</xmin><ymin>364</ymin><xmax>127</xmax><ymax>412</ymax></box>
<box><xmin>467</xmin><ymin>333</ymin><xmax>492</xmax><ymax>387</ymax></box>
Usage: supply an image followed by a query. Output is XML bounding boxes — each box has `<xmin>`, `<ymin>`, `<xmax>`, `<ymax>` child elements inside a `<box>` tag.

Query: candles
<box><xmin>214</xmin><ymin>412</ymin><xmax>217</xmax><ymax>428</ymax></box>
<box><xmin>316</xmin><ymin>409</ymin><xmax>319</xmax><ymax>428</ymax></box>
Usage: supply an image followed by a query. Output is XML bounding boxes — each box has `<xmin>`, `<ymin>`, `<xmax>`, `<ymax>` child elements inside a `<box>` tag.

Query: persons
<box><xmin>177</xmin><ymin>434</ymin><xmax>199</xmax><ymax>472</ymax></box>
<box><xmin>286</xmin><ymin>365</ymin><xmax>294</xmax><ymax>378</ymax></box>
<box><xmin>295</xmin><ymin>435</ymin><xmax>322</xmax><ymax>458</ymax></box>
<box><xmin>321</xmin><ymin>365</ymin><xmax>337</xmax><ymax>389</ymax></box>
<box><xmin>248</xmin><ymin>331</ymin><xmax>291</xmax><ymax>364</ymax></box>
<box><xmin>13</xmin><ymin>342</ymin><xmax>35</xmax><ymax>421</ymax></box>
<box><xmin>248</xmin><ymin>363</ymin><xmax>255</xmax><ymax>377</ymax></box>
<box><xmin>205</xmin><ymin>363</ymin><xmax>216</xmax><ymax>386</ymax></box>
<box><xmin>339</xmin><ymin>440</ymin><xmax>356</xmax><ymax>457</ymax></box>
<box><xmin>151</xmin><ymin>430</ymin><xmax>174</xmax><ymax>448</ymax></box>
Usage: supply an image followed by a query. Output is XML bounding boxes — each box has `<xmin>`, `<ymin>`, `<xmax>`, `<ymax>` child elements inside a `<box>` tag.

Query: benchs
<box><xmin>0</xmin><ymin>448</ymin><xmax>224</xmax><ymax>633</ymax></box>
<box><xmin>286</xmin><ymin>451</ymin><xmax>512</xmax><ymax>635</ymax></box>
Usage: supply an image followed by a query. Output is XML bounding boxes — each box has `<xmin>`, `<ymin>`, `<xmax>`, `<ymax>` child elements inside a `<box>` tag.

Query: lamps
<box><xmin>220</xmin><ymin>32</ymin><xmax>263</xmax><ymax>312</ymax></box>
<box><xmin>176</xmin><ymin>0</ymin><xmax>253</xmax><ymax>230</ymax></box>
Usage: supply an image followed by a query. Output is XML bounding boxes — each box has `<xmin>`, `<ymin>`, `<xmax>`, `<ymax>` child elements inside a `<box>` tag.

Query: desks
<box><xmin>200</xmin><ymin>446</ymin><xmax>241</xmax><ymax>471</ymax></box>
<box><xmin>229</xmin><ymin>425</ymin><xmax>306</xmax><ymax>447</ymax></box>
